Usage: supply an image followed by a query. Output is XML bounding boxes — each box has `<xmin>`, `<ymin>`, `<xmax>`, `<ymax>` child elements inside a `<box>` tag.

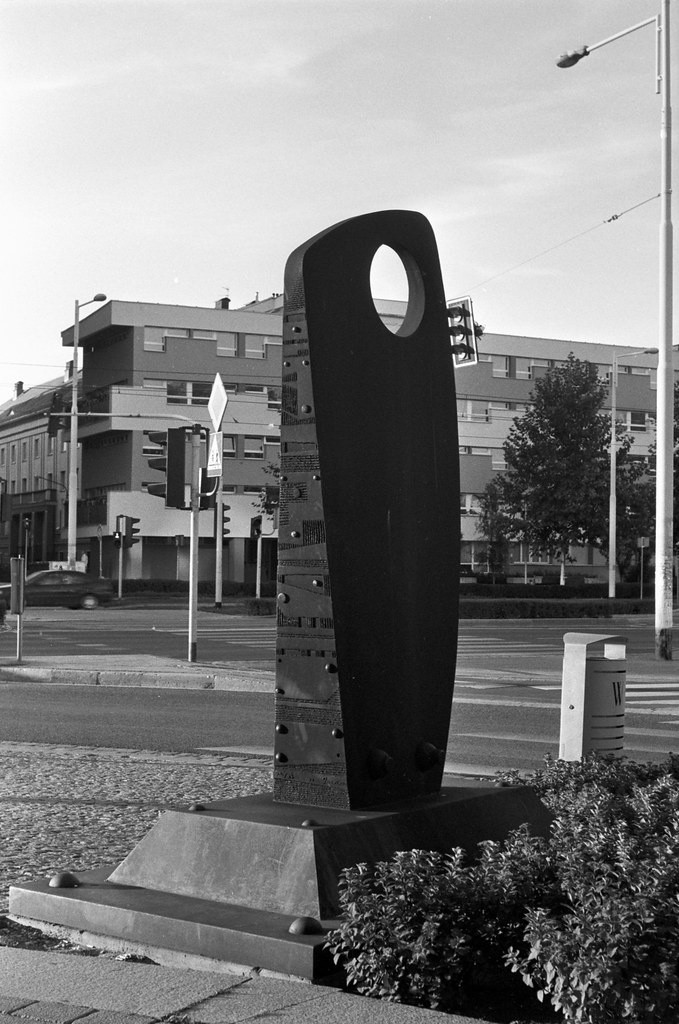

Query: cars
<box><xmin>0</xmin><ymin>570</ymin><xmax>115</xmax><ymax>610</ymax></box>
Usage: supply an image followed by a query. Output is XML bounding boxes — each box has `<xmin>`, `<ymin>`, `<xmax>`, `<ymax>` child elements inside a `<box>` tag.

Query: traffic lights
<box><xmin>445</xmin><ymin>296</ymin><xmax>484</xmax><ymax>369</ymax></box>
<box><xmin>113</xmin><ymin>532</ymin><xmax>121</xmax><ymax>548</ymax></box>
<box><xmin>250</xmin><ymin>518</ymin><xmax>261</xmax><ymax>540</ymax></box>
<box><xmin>116</xmin><ymin>514</ymin><xmax>140</xmax><ymax>549</ymax></box>
<box><xmin>147</xmin><ymin>427</ymin><xmax>187</xmax><ymax>510</ymax></box>
<box><xmin>222</xmin><ymin>504</ymin><xmax>230</xmax><ymax>536</ymax></box>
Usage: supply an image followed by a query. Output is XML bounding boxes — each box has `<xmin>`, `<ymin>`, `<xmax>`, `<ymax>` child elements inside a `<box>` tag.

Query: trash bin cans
<box><xmin>559</xmin><ymin>631</ymin><xmax>630</xmax><ymax>764</ymax></box>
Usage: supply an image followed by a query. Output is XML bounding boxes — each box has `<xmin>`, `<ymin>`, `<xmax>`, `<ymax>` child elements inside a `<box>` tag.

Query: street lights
<box><xmin>557</xmin><ymin>1</ymin><xmax>674</xmax><ymax>660</ymax></box>
<box><xmin>34</xmin><ymin>474</ymin><xmax>67</xmax><ymax>528</ymax></box>
<box><xmin>67</xmin><ymin>293</ymin><xmax>107</xmax><ymax>570</ymax></box>
<box><xmin>609</xmin><ymin>348</ymin><xmax>659</xmax><ymax>598</ymax></box>
